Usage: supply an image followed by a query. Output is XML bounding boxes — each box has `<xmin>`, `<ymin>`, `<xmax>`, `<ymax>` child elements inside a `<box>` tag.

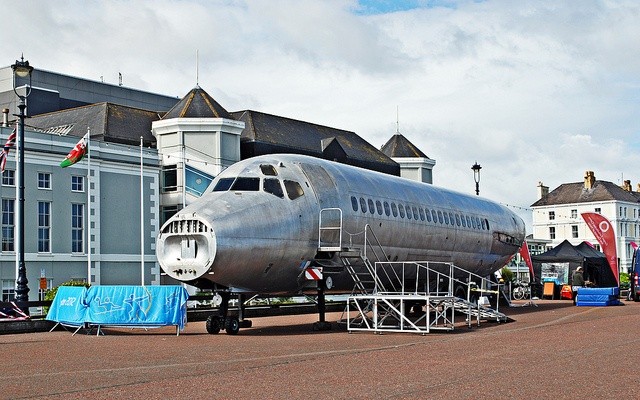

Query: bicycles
<box><xmin>513</xmin><ymin>278</ymin><xmax>528</xmax><ymax>300</ymax></box>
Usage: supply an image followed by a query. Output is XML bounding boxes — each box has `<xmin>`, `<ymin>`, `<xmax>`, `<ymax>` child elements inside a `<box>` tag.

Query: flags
<box><xmin>60</xmin><ymin>133</ymin><xmax>89</xmax><ymax>168</ymax></box>
<box><xmin>0</xmin><ymin>128</ymin><xmax>16</xmax><ymax>173</ymax></box>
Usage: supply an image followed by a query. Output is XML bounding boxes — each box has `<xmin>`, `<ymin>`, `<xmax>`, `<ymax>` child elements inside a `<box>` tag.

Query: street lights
<box><xmin>471</xmin><ymin>160</ymin><xmax>482</xmax><ymax>195</ymax></box>
<box><xmin>11</xmin><ymin>60</ymin><xmax>34</xmax><ymax>314</ymax></box>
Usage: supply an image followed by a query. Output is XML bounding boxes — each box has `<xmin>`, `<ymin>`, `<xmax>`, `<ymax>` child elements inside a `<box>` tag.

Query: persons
<box><xmin>570</xmin><ymin>265</ymin><xmax>586</xmax><ymax>306</ymax></box>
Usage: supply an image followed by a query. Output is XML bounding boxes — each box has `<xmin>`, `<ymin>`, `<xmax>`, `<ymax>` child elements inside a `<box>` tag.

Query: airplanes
<box><xmin>156</xmin><ymin>153</ymin><xmax>525</xmax><ymax>334</ymax></box>
